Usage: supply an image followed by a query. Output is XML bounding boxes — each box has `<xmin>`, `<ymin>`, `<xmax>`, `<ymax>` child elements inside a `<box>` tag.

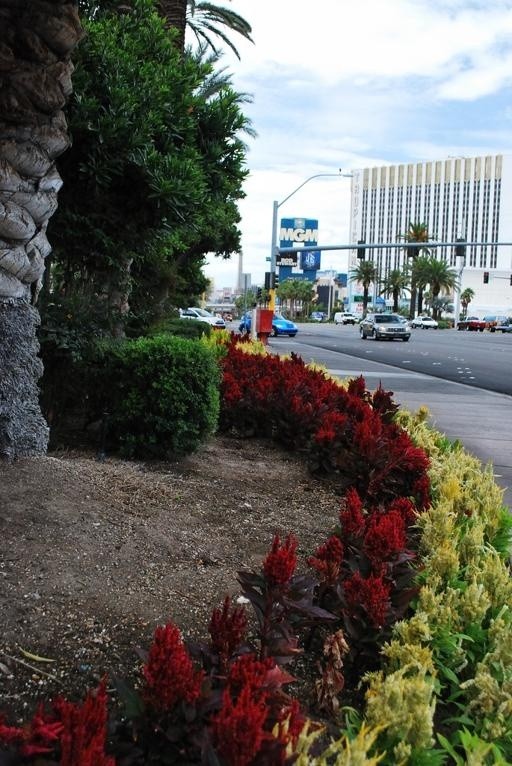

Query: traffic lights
<box><xmin>483</xmin><ymin>272</ymin><xmax>489</xmax><ymax>284</ymax></box>
<box><xmin>270</xmin><ymin>272</ymin><xmax>280</xmax><ymax>290</ymax></box>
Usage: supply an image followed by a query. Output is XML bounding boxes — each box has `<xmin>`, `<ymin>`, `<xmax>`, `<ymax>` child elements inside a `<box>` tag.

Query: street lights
<box><xmin>268</xmin><ymin>174</ymin><xmax>353</xmax><ymax>315</ymax></box>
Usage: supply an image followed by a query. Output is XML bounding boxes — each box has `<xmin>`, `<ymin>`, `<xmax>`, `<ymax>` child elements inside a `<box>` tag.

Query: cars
<box><xmin>174</xmin><ymin>307</ymin><xmax>226</xmax><ymax>331</ymax></box>
<box><xmin>238</xmin><ymin>311</ymin><xmax>298</xmax><ymax>337</ymax></box>
<box><xmin>358</xmin><ymin>312</ymin><xmax>439</xmax><ymax>342</ymax></box>
<box><xmin>456</xmin><ymin>316</ymin><xmax>512</xmax><ymax>333</ymax></box>
<box><xmin>223</xmin><ymin>313</ymin><xmax>233</xmax><ymax>322</ymax></box>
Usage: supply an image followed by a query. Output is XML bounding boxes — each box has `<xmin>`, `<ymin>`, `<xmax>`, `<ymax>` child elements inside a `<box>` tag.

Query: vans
<box><xmin>334</xmin><ymin>312</ymin><xmax>356</xmax><ymax>326</ymax></box>
